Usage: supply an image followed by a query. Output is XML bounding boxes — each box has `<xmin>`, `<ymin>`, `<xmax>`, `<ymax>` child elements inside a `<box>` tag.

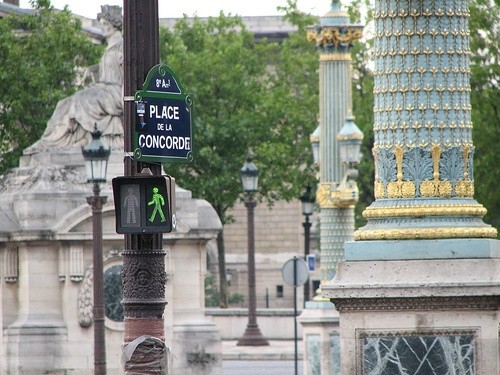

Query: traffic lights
<box><xmin>112</xmin><ymin>174</ymin><xmax>175</xmax><ymax>234</ymax></box>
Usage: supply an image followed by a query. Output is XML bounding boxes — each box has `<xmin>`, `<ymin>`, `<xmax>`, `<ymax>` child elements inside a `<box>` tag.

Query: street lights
<box><xmin>299</xmin><ymin>182</ymin><xmax>316</xmax><ymax>309</ymax></box>
<box><xmin>297</xmin><ymin>0</ymin><xmax>365</xmax><ymax>375</ymax></box>
<box><xmin>234</xmin><ymin>157</ymin><xmax>269</xmax><ymax>347</ymax></box>
<box><xmin>80</xmin><ymin>123</ymin><xmax>113</xmax><ymax>374</ymax></box>
